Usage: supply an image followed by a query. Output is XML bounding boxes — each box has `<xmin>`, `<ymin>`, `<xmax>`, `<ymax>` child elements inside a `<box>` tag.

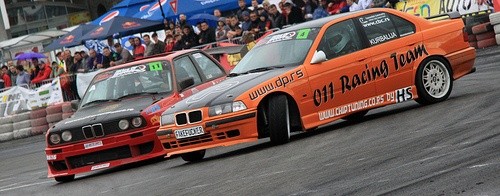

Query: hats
<box><xmin>113</xmin><ymin>43</ymin><xmax>120</xmax><ymax>47</ymax></box>
<box><xmin>241</xmin><ymin>10</ymin><xmax>249</xmax><ymax>16</ymax></box>
<box><xmin>37</xmin><ymin>60</ymin><xmax>44</xmax><ymax>65</ymax></box>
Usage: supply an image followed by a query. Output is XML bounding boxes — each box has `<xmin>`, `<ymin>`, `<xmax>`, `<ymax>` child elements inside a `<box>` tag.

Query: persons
<box><xmin>0</xmin><ymin>0</ymin><xmax>400</xmax><ymax>96</ymax></box>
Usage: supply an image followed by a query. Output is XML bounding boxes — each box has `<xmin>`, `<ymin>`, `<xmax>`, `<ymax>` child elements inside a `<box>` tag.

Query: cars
<box><xmin>44</xmin><ymin>43</ymin><xmax>249</xmax><ymax>184</ymax></box>
<box><xmin>156</xmin><ymin>8</ymin><xmax>478</xmax><ymax>163</ymax></box>
